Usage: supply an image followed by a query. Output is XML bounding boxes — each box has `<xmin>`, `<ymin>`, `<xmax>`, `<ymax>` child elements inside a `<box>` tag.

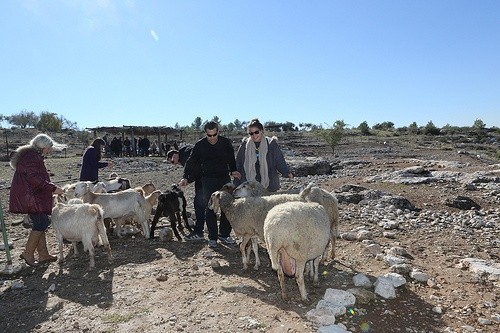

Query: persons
<box><xmin>80</xmin><ymin>138</ymin><xmax>113</xmax><ymax>182</ymax></box>
<box><xmin>233</xmin><ymin>119</ymin><xmax>294</xmax><ymax>192</ymax></box>
<box><xmin>166</xmin><ymin>144</ymin><xmax>225</xmax><ymax>241</ymax></box>
<box><xmin>8</xmin><ymin>133</ymin><xmax>64</xmax><ymax>267</ymax></box>
<box><xmin>177</xmin><ymin>121</ymin><xmax>241</xmax><ymax>248</ymax></box>
<box><xmin>102</xmin><ymin>133</ymin><xmax>195</xmax><ymax>158</ymax></box>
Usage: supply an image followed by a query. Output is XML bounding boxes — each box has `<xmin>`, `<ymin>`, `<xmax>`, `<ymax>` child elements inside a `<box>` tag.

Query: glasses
<box><xmin>249</xmin><ymin>129</ymin><xmax>261</xmax><ymax>135</ymax></box>
<box><xmin>206</xmin><ymin>132</ymin><xmax>218</xmax><ymax>137</ymax></box>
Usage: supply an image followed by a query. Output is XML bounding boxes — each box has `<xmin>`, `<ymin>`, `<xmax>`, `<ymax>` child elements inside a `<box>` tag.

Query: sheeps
<box><xmin>263</xmin><ymin>201</ymin><xmax>330</xmax><ymax>305</ymax></box>
<box><xmin>59</xmin><ymin>172</ymin><xmax>193</xmax><ymax>241</ymax></box>
<box><xmin>208</xmin><ymin>182</ymin><xmax>314</xmax><ymax>271</ymax></box>
<box><xmin>51</xmin><ymin>194</ymin><xmax>115</xmax><ymax>272</ymax></box>
<box><xmin>232</xmin><ymin>178</ymin><xmax>340</xmax><ymax>266</ymax></box>
<box><xmin>219</xmin><ymin>183</ymin><xmax>251</xmax><ymax>261</ymax></box>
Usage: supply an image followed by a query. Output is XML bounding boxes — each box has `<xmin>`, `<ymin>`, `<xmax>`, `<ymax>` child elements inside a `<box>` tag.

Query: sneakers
<box><xmin>208</xmin><ymin>238</ymin><xmax>218</xmax><ymax>248</ymax></box>
<box><xmin>217</xmin><ymin>234</ymin><xmax>235</xmax><ymax>243</ymax></box>
<box><xmin>184</xmin><ymin>232</ymin><xmax>204</xmax><ymax>241</ymax></box>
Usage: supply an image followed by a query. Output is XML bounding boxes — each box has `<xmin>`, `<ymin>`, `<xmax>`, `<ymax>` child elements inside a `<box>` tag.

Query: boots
<box><xmin>20</xmin><ymin>230</ymin><xmax>43</xmax><ymax>266</ymax></box>
<box><xmin>36</xmin><ymin>234</ymin><xmax>57</xmax><ymax>262</ymax></box>
